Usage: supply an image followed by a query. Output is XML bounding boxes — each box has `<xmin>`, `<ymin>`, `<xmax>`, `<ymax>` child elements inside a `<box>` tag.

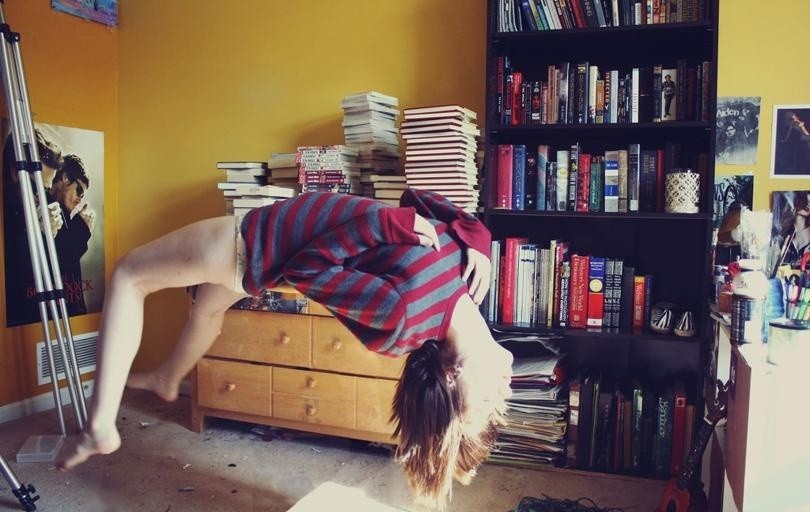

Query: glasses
<box><xmin>75</xmin><ymin>179</ymin><xmax>83</xmax><ymax>198</ymax></box>
<box><xmin>30</xmin><ymin>176</ymin><xmax>48</xmax><ymax>198</ymax></box>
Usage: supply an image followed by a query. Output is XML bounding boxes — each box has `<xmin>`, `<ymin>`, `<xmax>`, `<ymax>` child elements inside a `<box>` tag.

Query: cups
<box><xmin>767</xmin><ymin>318</ymin><xmax>810</xmax><ymax>365</ymax></box>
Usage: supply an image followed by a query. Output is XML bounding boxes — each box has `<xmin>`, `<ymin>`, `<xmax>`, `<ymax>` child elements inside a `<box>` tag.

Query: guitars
<box><xmin>656</xmin><ymin>379</ymin><xmax>731</xmax><ymax>512</ymax></box>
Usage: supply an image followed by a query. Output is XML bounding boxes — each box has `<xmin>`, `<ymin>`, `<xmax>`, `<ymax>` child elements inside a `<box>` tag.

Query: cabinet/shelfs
<box><xmin>187</xmin><ymin>284</ymin><xmax>411</xmax><ymax>459</ymax></box>
<box><xmin>699</xmin><ymin>308</ymin><xmax>809</xmax><ymax>512</ymax></box>
<box><xmin>482</xmin><ymin>0</ymin><xmax>720</xmax><ymax>489</ymax></box>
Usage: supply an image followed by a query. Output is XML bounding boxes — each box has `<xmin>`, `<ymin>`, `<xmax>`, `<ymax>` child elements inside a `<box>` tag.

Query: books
<box><xmin>489</xmin><ymin>236</ymin><xmax>654</xmax><ymax>330</ymax></box>
<box><xmin>496</xmin><ymin>335</ymin><xmax>698</xmax><ymax>483</ymax></box>
<box><xmin>216</xmin><ymin>90</ymin><xmax>483</xmax><ymax>226</ymax></box>
<box><xmin>487</xmin><ymin>0</ymin><xmax>721</xmax><ymax>32</ymax></box>
<box><xmin>487</xmin><ymin>38</ymin><xmax>714</xmax><ymax>128</ymax></box>
<box><xmin>486</xmin><ymin>141</ymin><xmax>708</xmax><ymax>216</ymax></box>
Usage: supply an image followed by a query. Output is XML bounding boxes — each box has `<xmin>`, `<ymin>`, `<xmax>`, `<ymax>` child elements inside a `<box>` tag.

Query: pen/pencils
<box><xmin>780</xmin><ymin>279</ymin><xmax>810</xmax><ymax>321</ymax></box>
<box><xmin>768</xmin><ymin>224</ymin><xmax>797</xmax><ymax>281</ymax></box>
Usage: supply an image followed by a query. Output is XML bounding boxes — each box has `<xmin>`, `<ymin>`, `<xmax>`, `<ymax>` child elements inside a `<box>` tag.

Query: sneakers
<box><xmin>650</xmin><ymin>299</ymin><xmax>676</xmax><ymax>334</ymax></box>
<box><xmin>673</xmin><ymin>309</ymin><xmax>694</xmax><ymax>336</ymax></box>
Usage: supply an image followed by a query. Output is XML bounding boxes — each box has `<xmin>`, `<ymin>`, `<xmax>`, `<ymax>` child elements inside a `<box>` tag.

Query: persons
<box><xmin>718</xmin><ymin>122</ymin><xmax>747</xmax><ymax>159</ymax></box>
<box><xmin>3</xmin><ymin>127</ymin><xmax>95</xmax><ymax>327</ymax></box>
<box><xmin>53</xmin><ymin>188</ymin><xmax>515</xmax><ymax>509</ymax></box>
